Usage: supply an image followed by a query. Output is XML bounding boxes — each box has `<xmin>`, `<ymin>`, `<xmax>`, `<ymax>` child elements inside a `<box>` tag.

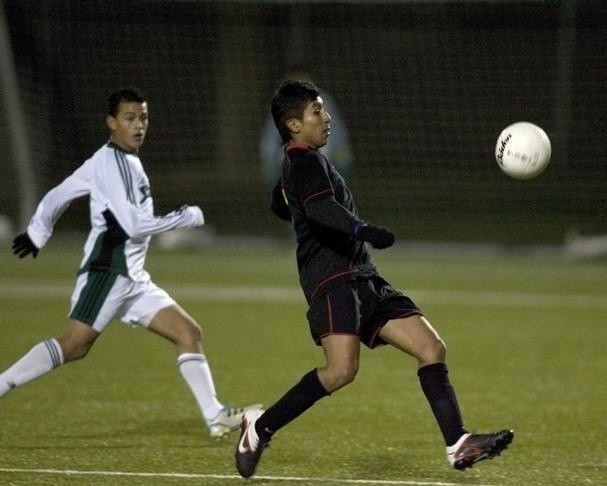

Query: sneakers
<box><xmin>444</xmin><ymin>429</ymin><xmax>513</xmax><ymax>472</ymax></box>
<box><xmin>209</xmin><ymin>403</ymin><xmax>269</xmax><ymax>477</ymax></box>
<box><xmin>12</xmin><ymin>233</ymin><xmax>39</xmax><ymax>259</ymax></box>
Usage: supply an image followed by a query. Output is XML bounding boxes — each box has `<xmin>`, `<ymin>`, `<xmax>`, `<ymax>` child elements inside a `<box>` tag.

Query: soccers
<box><xmin>495</xmin><ymin>121</ymin><xmax>551</xmax><ymax>181</ymax></box>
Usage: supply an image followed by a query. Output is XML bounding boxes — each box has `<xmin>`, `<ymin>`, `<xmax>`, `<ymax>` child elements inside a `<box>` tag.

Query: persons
<box><xmin>0</xmin><ymin>90</ymin><xmax>264</xmax><ymax>438</ymax></box>
<box><xmin>235</xmin><ymin>80</ymin><xmax>513</xmax><ymax>479</ymax></box>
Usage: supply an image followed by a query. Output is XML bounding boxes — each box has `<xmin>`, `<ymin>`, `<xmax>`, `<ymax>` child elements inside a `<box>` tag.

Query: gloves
<box><xmin>355</xmin><ymin>220</ymin><xmax>398</xmax><ymax>252</ymax></box>
